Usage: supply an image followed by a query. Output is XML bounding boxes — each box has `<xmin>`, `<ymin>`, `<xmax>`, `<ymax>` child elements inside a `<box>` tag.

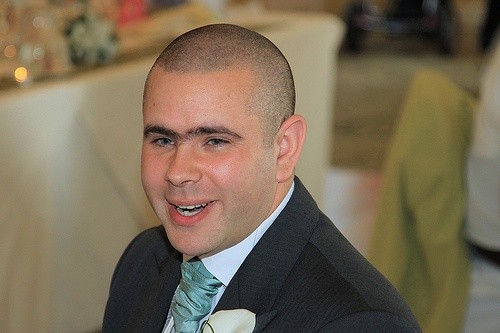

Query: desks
<box><xmin>0</xmin><ymin>10</ymin><xmax>346</xmax><ymax>333</ymax></box>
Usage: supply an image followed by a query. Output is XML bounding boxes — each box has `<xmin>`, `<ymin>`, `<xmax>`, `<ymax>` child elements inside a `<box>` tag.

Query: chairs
<box><xmin>365</xmin><ymin>67</ymin><xmax>500</xmax><ymax>333</ymax></box>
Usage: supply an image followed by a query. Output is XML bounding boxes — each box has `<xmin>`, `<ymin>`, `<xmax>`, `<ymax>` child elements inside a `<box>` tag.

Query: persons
<box><xmin>460</xmin><ymin>1</ymin><xmax>500</xmax><ymax>333</ymax></box>
<box><xmin>101</xmin><ymin>23</ymin><xmax>423</xmax><ymax>333</ymax></box>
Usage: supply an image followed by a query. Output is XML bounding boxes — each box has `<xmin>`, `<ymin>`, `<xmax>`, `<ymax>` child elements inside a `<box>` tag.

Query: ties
<box><xmin>170</xmin><ymin>256</ymin><xmax>223</xmax><ymax>333</ymax></box>
